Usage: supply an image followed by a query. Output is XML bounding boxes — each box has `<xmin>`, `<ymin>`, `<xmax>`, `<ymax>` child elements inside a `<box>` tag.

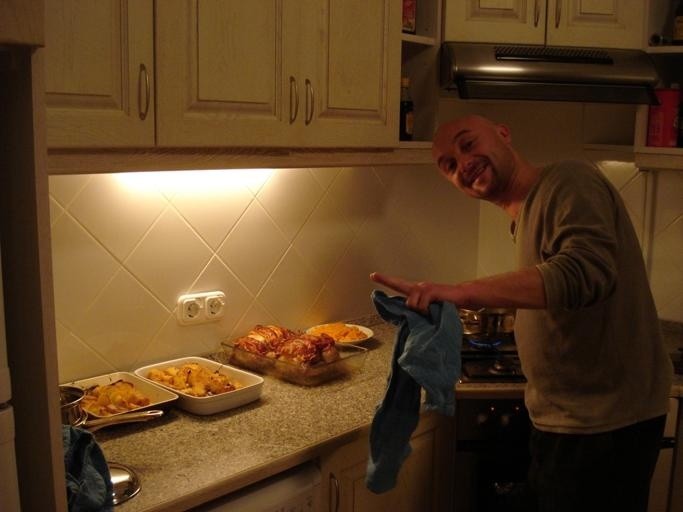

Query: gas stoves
<box><xmin>459</xmin><ymin>334</ymin><xmax>526</xmax><ymax>383</ymax></box>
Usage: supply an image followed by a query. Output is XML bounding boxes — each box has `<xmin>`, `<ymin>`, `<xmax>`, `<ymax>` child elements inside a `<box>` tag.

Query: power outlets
<box><xmin>178</xmin><ymin>291</ymin><xmax>228</xmax><ymax>326</ymax></box>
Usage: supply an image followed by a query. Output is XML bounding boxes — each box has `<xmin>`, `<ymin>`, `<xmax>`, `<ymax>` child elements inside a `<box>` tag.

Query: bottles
<box><xmin>398</xmin><ymin>76</ymin><xmax>416</xmax><ymax>141</ymax></box>
<box><xmin>647</xmin><ymin>82</ymin><xmax>680</xmax><ymax>147</ymax></box>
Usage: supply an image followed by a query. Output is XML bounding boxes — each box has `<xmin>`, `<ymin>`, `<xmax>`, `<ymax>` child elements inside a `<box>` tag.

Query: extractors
<box><xmin>437</xmin><ymin>43</ymin><xmax>663</xmax><ymax>107</ymax></box>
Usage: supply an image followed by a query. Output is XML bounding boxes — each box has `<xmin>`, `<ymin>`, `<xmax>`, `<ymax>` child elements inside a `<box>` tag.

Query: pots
<box><xmin>454</xmin><ymin>304</ymin><xmax>511</xmax><ymax>346</ymax></box>
<box><xmin>56</xmin><ymin>386</ymin><xmax>163</xmax><ymax>436</ymax></box>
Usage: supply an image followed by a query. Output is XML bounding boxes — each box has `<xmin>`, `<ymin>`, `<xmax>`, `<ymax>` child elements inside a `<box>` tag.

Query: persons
<box><xmin>368</xmin><ymin>112</ymin><xmax>673</xmax><ymax>511</ymax></box>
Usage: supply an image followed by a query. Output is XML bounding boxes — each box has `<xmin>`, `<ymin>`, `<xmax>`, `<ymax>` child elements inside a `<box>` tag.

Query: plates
<box><xmin>62</xmin><ymin>371</ymin><xmax>180</xmax><ymax>419</ymax></box>
<box><xmin>305</xmin><ymin>323</ymin><xmax>373</xmax><ymax>343</ymax></box>
<box><xmin>220</xmin><ymin>332</ymin><xmax>369</xmax><ymax>387</ymax></box>
<box><xmin>134</xmin><ymin>355</ymin><xmax>265</xmax><ymax>415</ymax></box>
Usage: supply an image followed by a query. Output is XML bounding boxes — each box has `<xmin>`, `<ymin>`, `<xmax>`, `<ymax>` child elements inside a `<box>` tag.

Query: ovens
<box><xmin>443</xmin><ymin>400</ymin><xmax>540</xmax><ymax>512</ymax></box>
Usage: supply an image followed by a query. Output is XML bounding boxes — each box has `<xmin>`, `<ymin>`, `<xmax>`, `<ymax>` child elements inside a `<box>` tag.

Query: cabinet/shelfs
<box><xmin>154</xmin><ymin>1</ymin><xmax>403</xmax><ymax>170</ymax></box>
<box><xmin>442</xmin><ymin>0</ymin><xmax>670</xmax><ymax>49</ymax></box>
<box><xmin>198</xmin><ymin>409</ymin><xmax>443</xmax><ymax>512</ymax></box>
<box><xmin>399</xmin><ymin>0</ymin><xmax>443</xmax><ymax>164</ymax></box>
<box><xmin>0</xmin><ymin>0</ymin><xmax>156</xmax><ymax>173</ymax></box>
<box><xmin>575</xmin><ymin>144</ymin><xmax>683</xmax><ymax>324</ymax></box>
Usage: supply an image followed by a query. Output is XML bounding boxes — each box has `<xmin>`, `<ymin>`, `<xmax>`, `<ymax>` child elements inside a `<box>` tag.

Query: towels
<box><xmin>362</xmin><ymin>289</ymin><xmax>465</xmax><ymax>494</ymax></box>
